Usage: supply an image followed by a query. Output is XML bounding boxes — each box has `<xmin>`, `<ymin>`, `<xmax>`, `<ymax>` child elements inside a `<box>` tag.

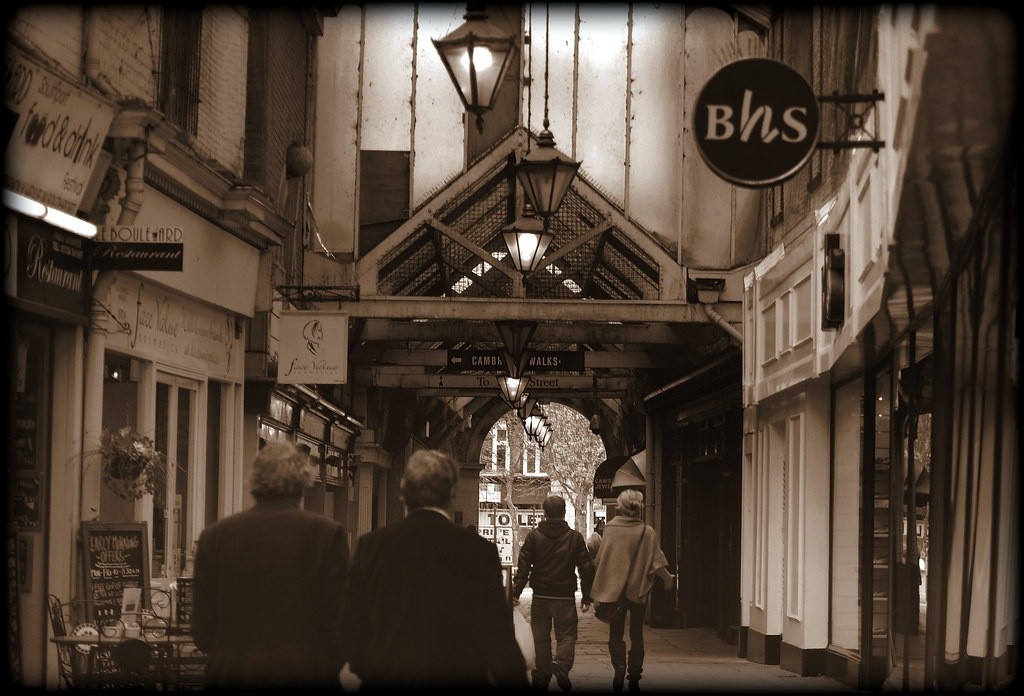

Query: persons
<box><xmin>187</xmin><ymin>441</ymin><xmax>350</xmax><ymax>696</ymax></box>
<box><xmin>346</xmin><ymin>450</ymin><xmax>526</xmax><ymax>696</ymax></box>
<box><xmin>510</xmin><ymin>496</ymin><xmax>594</xmax><ymax>691</ymax></box>
<box><xmin>590</xmin><ymin>490</ymin><xmax>676</xmax><ymax>692</ymax></box>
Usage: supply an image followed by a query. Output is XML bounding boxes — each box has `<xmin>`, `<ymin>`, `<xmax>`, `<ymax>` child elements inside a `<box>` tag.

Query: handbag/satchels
<box><xmin>594</xmin><ymin>599</ymin><xmax>623</xmax><ymax>623</ymax></box>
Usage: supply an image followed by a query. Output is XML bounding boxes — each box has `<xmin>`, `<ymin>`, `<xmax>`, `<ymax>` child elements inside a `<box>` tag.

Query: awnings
<box><xmin>594</xmin><ymin>450</ymin><xmax>646</xmax><ymax>498</ymax></box>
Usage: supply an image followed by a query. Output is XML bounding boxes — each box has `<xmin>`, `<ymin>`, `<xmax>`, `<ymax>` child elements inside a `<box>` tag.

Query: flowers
<box><xmin>68</xmin><ymin>422</ymin><xmax>182</xmax><ymax>504</ymax></box>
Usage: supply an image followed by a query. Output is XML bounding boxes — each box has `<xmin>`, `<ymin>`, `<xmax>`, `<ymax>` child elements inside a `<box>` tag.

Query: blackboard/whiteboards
<box><xmin>80</xmin><ymin>520</ymin><xmax>152</xmax><ymax>624</ymax></box>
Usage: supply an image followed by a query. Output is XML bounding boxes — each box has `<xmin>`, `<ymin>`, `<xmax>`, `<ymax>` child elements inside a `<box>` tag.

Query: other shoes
<box><xmin>551</xmin><ymin>661</ymin><xmax>572</xmax><ymax>691</ymax></box>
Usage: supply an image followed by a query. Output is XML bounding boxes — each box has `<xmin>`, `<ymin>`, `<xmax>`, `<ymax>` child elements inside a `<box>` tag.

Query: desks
<box><xmin>50</xmin><ymin>635</ymin><xmax>199</xmax><ymax>696</ymax></box>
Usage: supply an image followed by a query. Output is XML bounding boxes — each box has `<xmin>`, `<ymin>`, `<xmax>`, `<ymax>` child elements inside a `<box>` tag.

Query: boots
<box><xmin>626</xmin><ymin>650</ymin><xmax>645</xmax><ymax>693</ymax></box>
<box><xmin>607</xmin><ymin>640</ymin><xmax>627</xmax><ymax>691</ymax></box>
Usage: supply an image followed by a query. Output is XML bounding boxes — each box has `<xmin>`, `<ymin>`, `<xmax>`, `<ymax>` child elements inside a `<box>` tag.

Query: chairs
<box><xmin>45</xmin><ymin>587</ymin><xmax>207</xmax><ymax>696</ymax></box>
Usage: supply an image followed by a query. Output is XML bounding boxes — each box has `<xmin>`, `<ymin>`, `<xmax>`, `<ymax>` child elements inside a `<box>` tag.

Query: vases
<box><xmin>111</xmin><ymin>456</ymin><xmax>143</xmax><ymax>480</ymax></box>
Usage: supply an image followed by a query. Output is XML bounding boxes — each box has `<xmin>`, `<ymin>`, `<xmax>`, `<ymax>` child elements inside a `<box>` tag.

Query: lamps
<box><xmin>534</xmin><ymin>421</ymin><xmax>552</xmax><ymax>446</ymax></box>
<box><xmin>321</xmin><ymin>456</ymin><xmax>341</xmax><ymax>467</ymax></box>
<box><xmin>520</xmin><ymin>404</ymin><xmax>544</xmax><ymax>442</ymax></box>
<box><xmin>494</xmin><ymin>321</ymin><xmax>538</xmax><ymax>367</ymax></box>
<box><xmin>512</xmin><ymin>0</ymin><xmax>582</xmax><ymax>232</ymax></box>
<box><xmin>500</xmin><ymin>0</ymin><xmax>559</xmax><ymax>287</ymax></box>
<box><xmin>515</xmin><ymin>397</ymin><xmax>538</xmax><ymax>424</ymax></box>
<box><xmin>496</xmin><ymin>347</ymin><xmax>537</xmax><ymax>380</ymax></box>
<box><xmin>498</xmin><ymin>390</ymin><xmax>530</xmax><ymax>411</ymax></box>
<box><xmin>496</xmin><ymin>370</ymin><xmax>532</xmax><ymax>403</ymax></box>
<box><xmin>540</xmin><ymin>429</ymin><xmax>554</xmax><ymax>452</ymax></box>
<box><xmin>431</xmin><ymin>1</ymin><xmax>523</xmax><ymax>136</ymax></box>
<box><xmin>4</xmin><ymin>189</ymin><xmax>97</xmax><ymax>240</ymax></box>
<box><xmin>534</xmin><ymin>413</ymin><xmax>548</xmax><ymax>440</ymax></box>
